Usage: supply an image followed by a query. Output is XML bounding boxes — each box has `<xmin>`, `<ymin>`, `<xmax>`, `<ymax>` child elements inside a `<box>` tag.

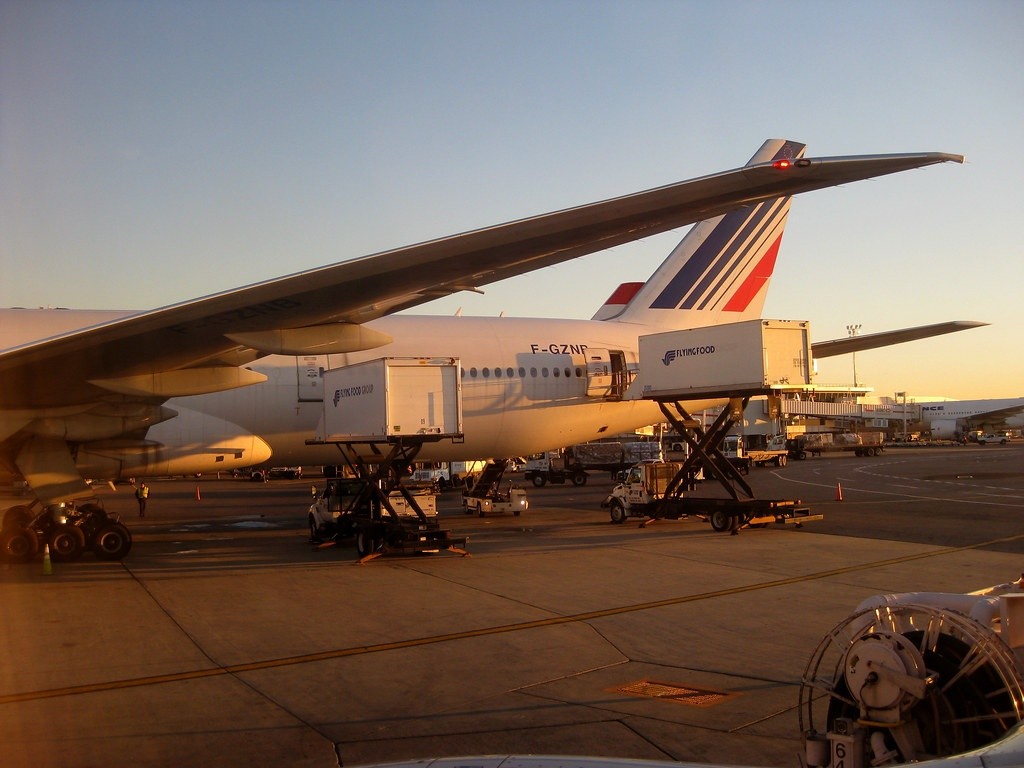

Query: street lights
<box><xmin>846</xmin><ymin>323</ymin><xmax>863</xmax><ymax>387</ymax></box>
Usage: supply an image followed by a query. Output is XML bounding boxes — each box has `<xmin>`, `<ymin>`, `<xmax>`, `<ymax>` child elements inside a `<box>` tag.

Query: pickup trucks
<box><xmin>978</xmin><ymin>434</ymin><xmax>1011</xmax><ymax>445</ymax></box>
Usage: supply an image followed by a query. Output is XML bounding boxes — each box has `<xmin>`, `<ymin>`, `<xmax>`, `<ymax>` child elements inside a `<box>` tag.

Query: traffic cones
<box><xmin>834</xmin><ymin>481</ymin><xmax>843</xmax><ymax>502</ymax></box>
<box><xmin>194</xmin><ymin>484</ymin><xmax>201</xmax><ymax>501</ymax></box>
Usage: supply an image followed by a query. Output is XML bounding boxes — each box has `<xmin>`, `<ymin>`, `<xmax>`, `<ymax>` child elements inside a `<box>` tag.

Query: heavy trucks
<box><xmin>523</xmin><ymin>441</ymin><xmax>666</xmax><ymax>487</ymax></box>
<box><xmin>764</xmin><ymin>435</ymin><xmax>887</xmax><ymax>460</ymax></box>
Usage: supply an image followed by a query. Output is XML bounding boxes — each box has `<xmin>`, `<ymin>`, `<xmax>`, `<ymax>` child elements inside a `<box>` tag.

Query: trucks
<box><xmin>600</xmin><ymin>457</ymin><xmax>825</xmax><ymax>536</ymax></box>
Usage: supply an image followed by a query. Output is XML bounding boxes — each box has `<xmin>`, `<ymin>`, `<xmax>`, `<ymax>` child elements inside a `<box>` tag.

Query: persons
<box><xmin>135</xmin><ymin>482</ymin><xmax>150</xmax><ymax>517</ymax></box>
<box><xmin>907</xmin><ymin>434</ymin><xmax>912</xmax><ymax>442</ymax></box>
<box><xmin>962</xmin><ymin>435</ymin><xmax>968</xmax><ymax>446</ymax></box>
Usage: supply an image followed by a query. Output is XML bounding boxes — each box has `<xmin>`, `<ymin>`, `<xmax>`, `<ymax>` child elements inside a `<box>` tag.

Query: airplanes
<box><xmin>0</xmin><ymin>139</ymin><xmax>995</xmax><ymax>565</ymax></box>
